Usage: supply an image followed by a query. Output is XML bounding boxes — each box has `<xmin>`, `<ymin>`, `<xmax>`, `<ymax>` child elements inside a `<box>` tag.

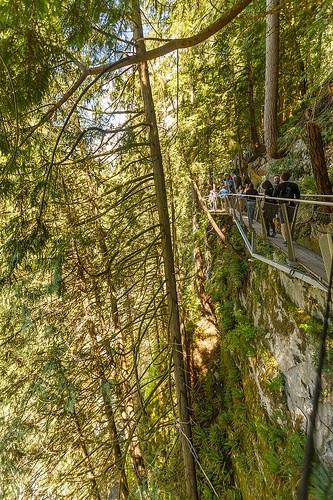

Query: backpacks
<box><xmin>280</xmin><ymin>183</ymin><xmax>293</xmax><ymax>203</ymax></box>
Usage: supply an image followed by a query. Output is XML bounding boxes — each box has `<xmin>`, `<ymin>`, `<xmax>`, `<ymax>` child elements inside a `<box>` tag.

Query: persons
<box><xmin>263</xmin><ymin>170</ymin><xmax>300</xmax><ymax>247</ymax></box>
<box><xmin>206</xmin><ymin>171</ymin><xmax>259</xmax><ymax>224</ymax></box>
